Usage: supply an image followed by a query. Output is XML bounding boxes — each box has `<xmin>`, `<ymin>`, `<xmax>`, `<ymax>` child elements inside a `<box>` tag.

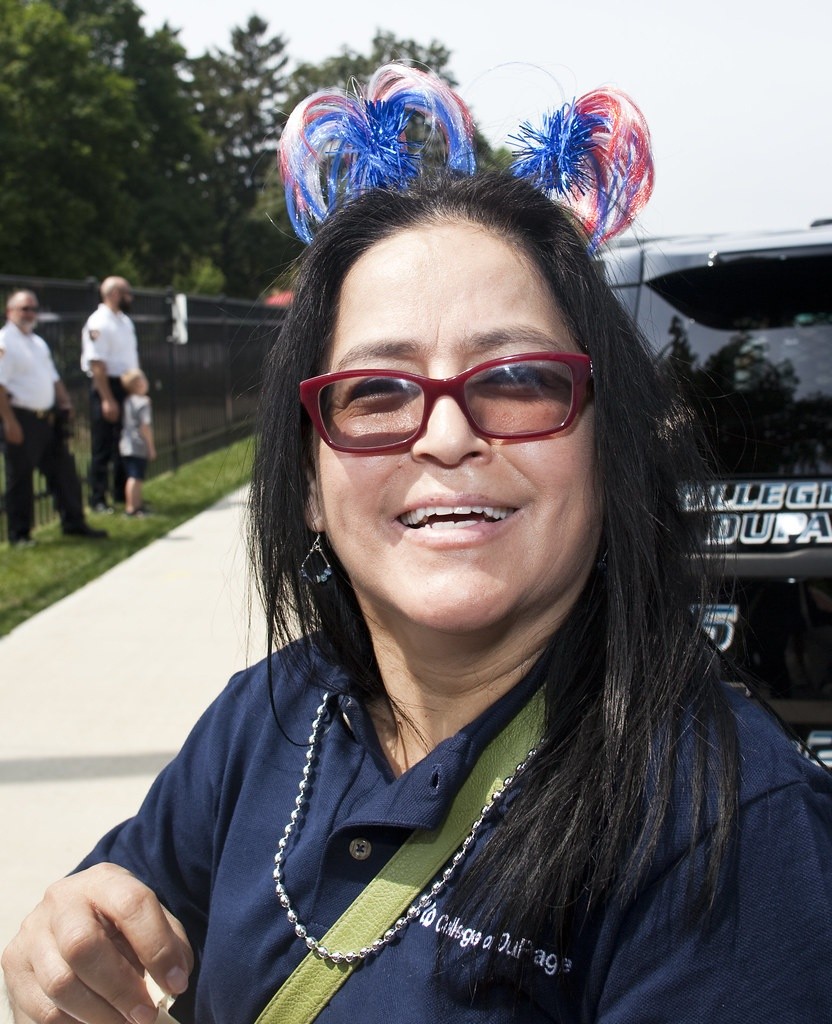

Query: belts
<box><xmin>14</xmin><ymin>405</ymin><xmax>57</xmax><ymax>419</ymax></box>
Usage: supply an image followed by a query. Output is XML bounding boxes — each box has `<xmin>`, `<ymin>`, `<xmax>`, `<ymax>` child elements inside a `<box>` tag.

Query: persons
<box><xmin>0</xmin><ymin>164</ymin><xmax>831</xmax><ymax>1024</ymax></box>
<box><xmin>80</xmin><ymin>276</ymin><xmax>158</xmax><ymax>518</ymax></box>
<box><xmin>0</xmin><ymin>289</ymin><xmax>109</xmax><ymax>548</ymax></box>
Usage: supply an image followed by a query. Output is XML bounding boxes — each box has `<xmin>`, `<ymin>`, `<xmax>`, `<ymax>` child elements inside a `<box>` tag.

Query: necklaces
<box><xmin>270</xmin><ymin>645</ymin><xmax>556</xmax><ymax>967</ymax></box>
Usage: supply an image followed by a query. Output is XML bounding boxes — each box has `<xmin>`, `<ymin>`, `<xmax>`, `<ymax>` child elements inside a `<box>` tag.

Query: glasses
<box><xmin>12</xmin><ymin>306</ymin><xmax>38</xmax><ymax>313</ymax></box>
<box><xmin>299</xmin><ymin>351</ymin><xmax>595</xmax><ymax>453</ymax></box>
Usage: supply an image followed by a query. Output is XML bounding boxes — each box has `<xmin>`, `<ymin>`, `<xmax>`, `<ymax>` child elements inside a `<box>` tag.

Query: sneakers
<box><xmin>10</xmin><ymin>539</ymin><xmax>37</xmax><ymax>548</ymax></box>
<box><xmin>63</xmin><ymin>523</ymin><xmax>107</xmax><ymax>539</ymax></box>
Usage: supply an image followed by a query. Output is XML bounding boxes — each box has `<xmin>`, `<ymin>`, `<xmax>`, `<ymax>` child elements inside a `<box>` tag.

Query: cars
<box><xmin>583</xmin><ymin>221</ymin><xmax>831</xmax><ymax>790</ymax></box>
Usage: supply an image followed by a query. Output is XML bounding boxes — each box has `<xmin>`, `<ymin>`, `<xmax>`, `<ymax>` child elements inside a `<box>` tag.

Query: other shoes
<box><xmin>92</xmin><ymin>502</ymin><xmax>114</xmax><ymax>515</ymax></box>
<box><xmin>121</xmin><ymin>508</ymin><xmax>155</xmax><ymax>519</ymax></box>
